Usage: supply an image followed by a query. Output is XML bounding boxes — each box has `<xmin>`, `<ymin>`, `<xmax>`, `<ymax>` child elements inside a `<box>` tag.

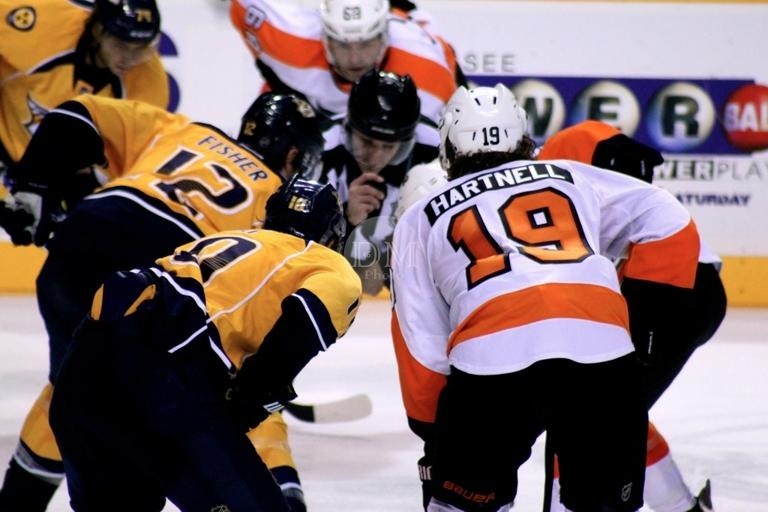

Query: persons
<box><xmin>0</xmin><ymin>4</ymin><xmax>173</xmax><ymax>184</ymax></box>
<box><xmin>3</xmin><ymin>90</ymin><xmax>331</xmax><ymax>510</ymax></box>
<box><xmin>296</xmin><ymin>68</ymin><xmax>457</xmax><ymax>283</ymax></box>
<box><xmin>224</xmin><ymin>0</ymin><xmax>460</xmax><ymax>177</ymax></box>
<box><xmin>530</xmin><ymin>116</ymin><xmax>727</xmax><ymax>508</ymax></box>
<box><xmin>386</xmin><ymin>86</ymin><xmax>699</xmax><ymax>510</ymax></box>
<box><xmin>45</xmin><ymin>175</ymin><xmax>361</xmax><ymax>509</ymax></box>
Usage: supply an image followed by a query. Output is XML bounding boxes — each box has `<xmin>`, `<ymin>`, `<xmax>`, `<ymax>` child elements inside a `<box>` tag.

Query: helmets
<box><xmin>438</xmin><ymin>82</ymin><xmax>535</xmax><ymax>181</ymax></box>
<box><xmin>235</xmin><ymin>91</ymin><xmax>334</xmax><ymax>182</ymax></box>
<box><xmin>94</xmin><ymin>1</ymin><xmax>160</xmax><ymax>41</ymax></box>
<box><xmin>265</xmin><ymin>177</ymin><xmax>343</xmax><ymax>246</ymax></box>
<box><xmin>344</xmin><ymin>66</ymin><xmax>421</xmax><ymax>143</ymax></box>
<box><xmin>320</xmin><ymin>0</ymin><xmax>394</xmax><ymax>67</ymax></box>
